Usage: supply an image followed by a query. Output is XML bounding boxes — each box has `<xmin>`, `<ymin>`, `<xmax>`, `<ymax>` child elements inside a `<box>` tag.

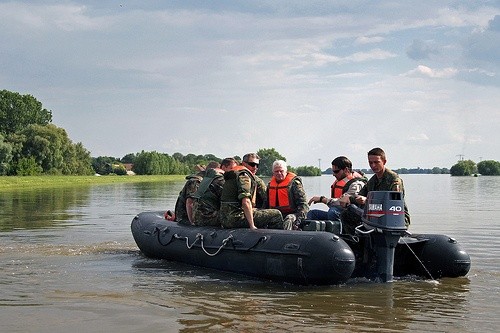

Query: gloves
<box><xmin>282</xmin><ymin>214</ymin><xmax>297</xmax><ymax>231</ymax></box>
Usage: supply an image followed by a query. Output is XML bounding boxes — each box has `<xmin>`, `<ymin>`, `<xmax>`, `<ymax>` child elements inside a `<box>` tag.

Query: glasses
<box><xmin>332</xmin><ymin>168</ymin><xmax>342</xmax><ymax>174</ymax></box>
<box><xmin>243</xmin><ymin>160</ymin><xmax>259</xmax><ymax>168</ymax></box>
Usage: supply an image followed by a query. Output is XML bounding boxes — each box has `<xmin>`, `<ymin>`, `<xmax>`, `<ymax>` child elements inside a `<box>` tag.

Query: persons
<box><xmin>221</xmin><ymin>153</ymin><xmax>283</xmax><ymax>230</ymax></box>
<box><xmin>192</xmin><ymin>158</ymin><xmax>239</xmax><ymax>227</ymax></box>
<box><xmin>307</xmin><ymin>156</ymin><xmax>368</xmax><ymax>234</ymax></box>
<box><xmin>175</xmin><ymin>162</ymin><xmax>266</xmax><ymax>225</ymax></box>
<box><xmin>262</xmin><ymin>160</ymin><xmax>306</xmax><ymax>230</ymax></box>
<box><xmin>340</xmin><ymin>148</ymin><xmax>410</xmax><ymax>237</ymax></box>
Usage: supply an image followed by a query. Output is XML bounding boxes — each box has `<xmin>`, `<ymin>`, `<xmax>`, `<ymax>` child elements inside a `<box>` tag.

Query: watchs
<box><xmin>320</xmin><ymin>196</ymin><xmax>325</xmax><ymax>202</ymax></box>
